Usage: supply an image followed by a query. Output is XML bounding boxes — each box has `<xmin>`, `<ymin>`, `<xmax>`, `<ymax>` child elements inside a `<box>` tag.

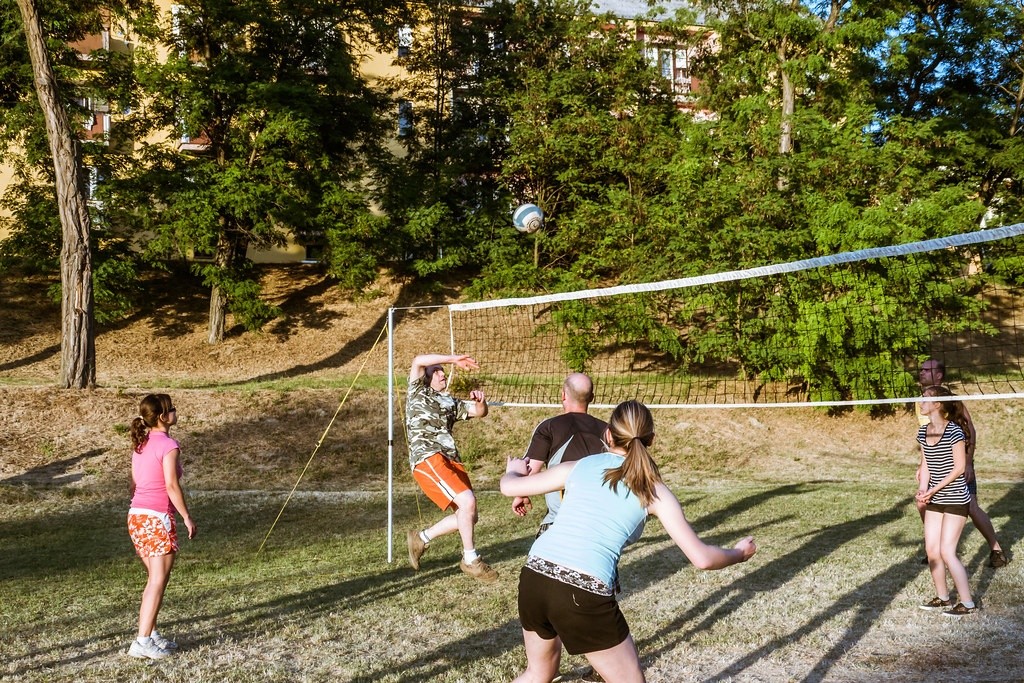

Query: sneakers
<box><xmin>581</xmin><ymin>669</ymin><xmax>605</xmax><ymax>683</ymax></box>
<box><xmin>920</xmin><ymin>556</ymin><xmax>929</xmax><ymax>565</ymax></box>
<box><xmin>407</xmin><ymin>528</ymin><xmax>430</xmax><ymax>570</ymax></box>
<box><xmin>551</xmin><ymin>671</ymin><xmax>563</xmax><ymax>683</ymax></box>
<box><xmin>989</xmin><ymin>548</ymin><xmax>1007</xmax><ymax>567</ymax></box>
<box><xmin>128</xmin><ymin>638</ymin><xmax>171</xmax><ymax>659</ymax></box>
<box><xmin>918</xmin><ymin>597</ymin><xmax>953</xmax><ymax>610</ymax></box>
<box><xmin>150</xmin><ymin>635</ymin><xmax>178</xmax><ymax>650</ymax></box>
<box><xmin>943</xmin><ymin>603</ymin><xmax>978</xmax><ymax>616</ymax></box>
<box><xmin>460</xmin><ymin>554</ymin><xmax>499</xmax><ymax>581</ymax></box>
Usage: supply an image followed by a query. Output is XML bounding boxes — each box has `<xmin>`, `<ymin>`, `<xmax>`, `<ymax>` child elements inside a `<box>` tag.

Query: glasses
<box><xmin>921</xmin><ymin>368</ymin><xmax>939</xmax><ymax>373</ymax></box>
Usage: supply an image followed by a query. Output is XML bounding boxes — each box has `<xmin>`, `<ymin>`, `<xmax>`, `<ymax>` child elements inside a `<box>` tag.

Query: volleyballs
<box><xmin>513</xmin><ymin>203</ymin><xmax>544</xmax><ymax>234</ymax></box>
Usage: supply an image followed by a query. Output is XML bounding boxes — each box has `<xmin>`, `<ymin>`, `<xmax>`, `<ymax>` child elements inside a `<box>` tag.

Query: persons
<box><xmin>915</xmin><ymin>386</ymin><xmax>979</xmax><ymax>616</ymax></box>
<box><xmin>406</xmin><ymin>354</ymin><xmax>499</xmax><ymax>580</ymax></box>
<box><xmin>127</xmin><ymin>393</ymin><xmax>197</xmax><ymax>658</ymax></box>
<box><xmin>915</xmin><ymin>358</ymin><xmax>1006</xmax><ymax>568</ymax></box>
<box><xmin>511</xmin><ymin>372</ymin><xmax>620</xmax><ymax>683</ymax></box>
<box><xmin>500</xmin><ymin>400</ymin><xmax>757</xmax><ymax>683</ymax></box>
